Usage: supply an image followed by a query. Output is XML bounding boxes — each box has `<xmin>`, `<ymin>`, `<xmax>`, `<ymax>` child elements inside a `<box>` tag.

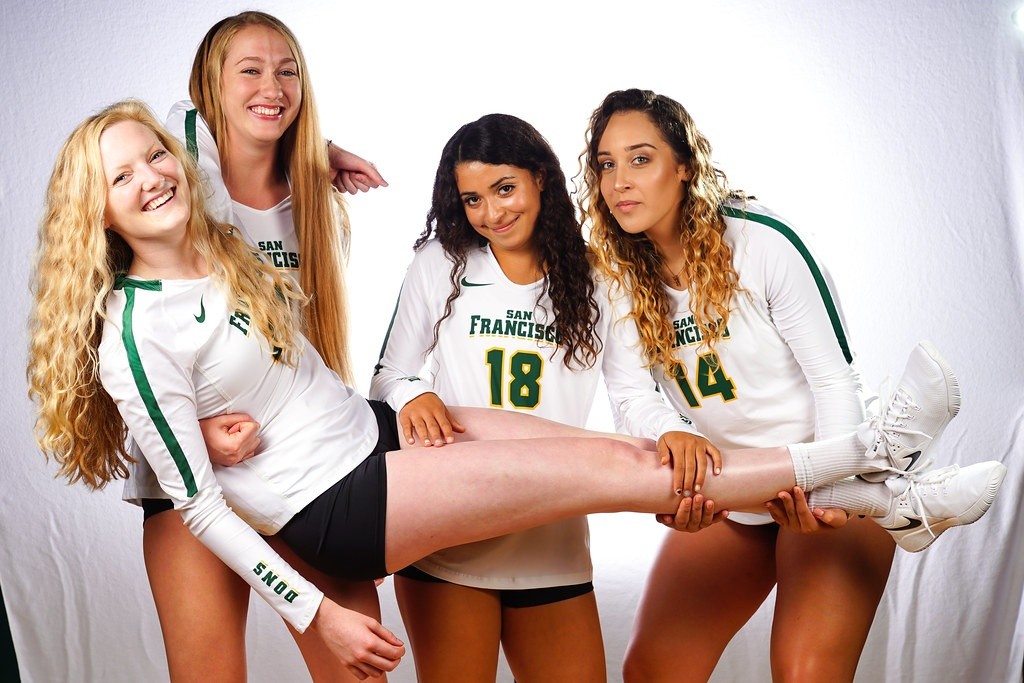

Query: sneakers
<box><xmin>858</xmin><ymin>342</ymin><xmax>960</xmax><ymax>483</ymax></box>
<box><xmin>871</xmin><ymin>461</ymin><xmax>1008</xmax><ymax>553</ymax></box>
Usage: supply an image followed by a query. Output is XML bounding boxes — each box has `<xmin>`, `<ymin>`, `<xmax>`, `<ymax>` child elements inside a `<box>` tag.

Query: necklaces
<box><xmin>661</xmin><ymin>256</ymin><xmax>687</xmax><ymax>287</ymax></box>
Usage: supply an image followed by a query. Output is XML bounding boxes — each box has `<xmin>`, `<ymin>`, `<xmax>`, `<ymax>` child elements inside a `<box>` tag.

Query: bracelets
<box><xmin>325</xmin><ymin>137</ymin><xmax>333</xmax><ymax>151</ymax></box>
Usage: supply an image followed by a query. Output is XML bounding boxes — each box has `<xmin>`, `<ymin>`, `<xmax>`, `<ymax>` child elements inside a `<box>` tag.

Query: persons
<box><xmin>581</xmin><ymin>90</ymin><xmax>899</xmax><ymax>683</ymax></box>
<box><xmin>368</xmin><ymin>112</ymin><xmax>722</xmax><ymax>683</ymax></box>
<box><xmin>124</xmin><ymin>10</ymin><xmax>393</xmax><ymax>683</ymax></box>
<box><xmin>29</xmin><ymin>101</ymin><xmax>1011</xmax><ymax>683</ymax></box>
